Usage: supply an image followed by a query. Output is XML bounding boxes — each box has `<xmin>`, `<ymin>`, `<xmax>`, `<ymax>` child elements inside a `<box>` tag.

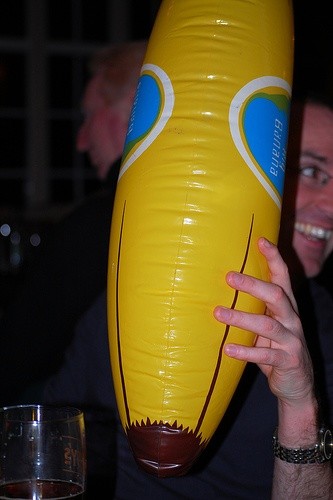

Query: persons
<box><xmin>1</xmin><ymin>42</ymin><xmax>149</xmax><ymax>404</ymax></box>
<box><xmin>42</xmin><ymin>88</ymin><xmax>333</xmax><ymax>500</ymax></box>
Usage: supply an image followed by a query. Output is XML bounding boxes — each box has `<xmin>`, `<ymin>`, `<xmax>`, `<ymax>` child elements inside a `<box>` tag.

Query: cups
<box><xmin>0</xmin><ymin>404</ymin><xmax>87</xmax><ymax>500</ymax></box>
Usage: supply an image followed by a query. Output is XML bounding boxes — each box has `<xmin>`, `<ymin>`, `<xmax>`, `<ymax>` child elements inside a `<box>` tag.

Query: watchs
<box><xmin>272</xmin><ymin>420</ymin><xmax>333</xmax><ymax>464</ymax></box>
